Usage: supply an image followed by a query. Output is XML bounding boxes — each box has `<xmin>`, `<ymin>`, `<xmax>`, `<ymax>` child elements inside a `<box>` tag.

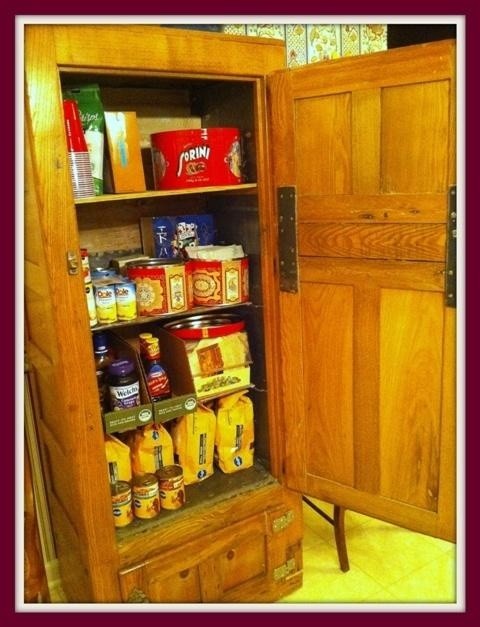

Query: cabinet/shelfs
<box><xmin>23</xmin><ymin>24</ymin><xmax>458</xmax><ymax>605</ymax></box>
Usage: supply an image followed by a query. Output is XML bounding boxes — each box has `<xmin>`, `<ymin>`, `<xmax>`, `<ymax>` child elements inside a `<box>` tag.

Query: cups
<box><xmin>63</xmin><ymin>98</ymin><xmax>92</xmax><ymax>197</ymax></box>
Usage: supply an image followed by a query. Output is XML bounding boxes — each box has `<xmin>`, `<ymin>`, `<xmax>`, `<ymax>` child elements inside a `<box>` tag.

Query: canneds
<box><xmin>131</xmin><ymin>473</ymin><xmax>161</xmax><ymax>519</ymax></box>
<box><xmin>107</xmin><ymin>360</ymin><xmax>141</xmax><ymax>411</ymax></box>
<box><xmin>80</xmin><ymin>249</ymin><xmax>137</xmax><ymax>327</ymax></box>
<box><xmin>109</xmin><ymin>480</ymin><xmax>133</xmax><ymax>528</ymax></box>
<box><xmin>154</xmin><ymin>466</ymin><xmax>186</xmax><ymax>511</ymax></box>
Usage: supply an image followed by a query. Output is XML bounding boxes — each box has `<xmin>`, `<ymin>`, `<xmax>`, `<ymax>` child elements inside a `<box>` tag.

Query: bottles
<box><xmin>139</xmin><ymin>333</ymin><xmax>172</xmax><ymax>403</ymax></box>
<box><xmin>90</xmin><ymin>333</ymin><xmax>115</xmax><ymax>414</ymax></box>
